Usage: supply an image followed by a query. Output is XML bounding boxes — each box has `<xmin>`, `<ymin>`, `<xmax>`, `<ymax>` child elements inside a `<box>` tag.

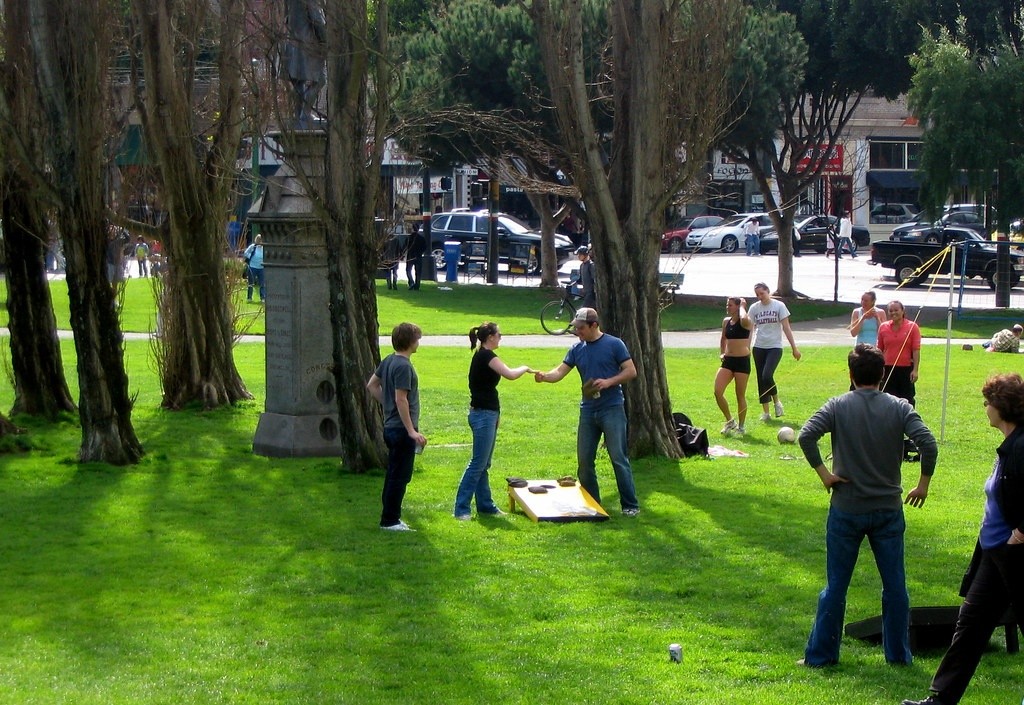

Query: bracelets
<box><xmin>1011</xmin><ymin>528</ymin><xmax>1024</xmax><ymax>543</ymax></box>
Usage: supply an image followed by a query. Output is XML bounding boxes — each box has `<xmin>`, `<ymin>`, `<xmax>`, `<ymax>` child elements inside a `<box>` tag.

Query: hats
<box><xmin>578</xmin><ymin>246</ymin><xmax>589</xmax><ymax>253</ymax></box>
<box><xmin>570</xmin><ymin>307</ymin><xmax>599</xmax><ymax>329</ymax></box>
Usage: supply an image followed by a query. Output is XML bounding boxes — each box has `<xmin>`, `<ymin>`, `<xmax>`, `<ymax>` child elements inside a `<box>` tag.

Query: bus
<box><xmin>895</xmin><ymin>203</ymin><xmax>998</xmax><ymax>230</ymax></box>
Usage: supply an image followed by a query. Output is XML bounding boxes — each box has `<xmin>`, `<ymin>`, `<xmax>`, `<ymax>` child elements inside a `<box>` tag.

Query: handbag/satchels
<box><xmin>242</xmin><ymin>258</ymin><xmax>250</xmax><ymax>279</ymax></box>
<box><xmin>676</xmin><ymin>423</ymin><xmax>709</xmax><ymax>457</ymax></box>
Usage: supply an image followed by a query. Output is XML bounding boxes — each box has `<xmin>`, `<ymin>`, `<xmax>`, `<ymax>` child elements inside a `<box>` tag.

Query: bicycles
<box><xmin>540</xmin><ymin>281</ymin><xmax>585</xmax><ymax>336</ymax></box>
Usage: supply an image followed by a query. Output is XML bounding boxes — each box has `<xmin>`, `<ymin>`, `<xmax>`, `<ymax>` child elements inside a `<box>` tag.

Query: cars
<box><xmin>889</xmin><ymin>209</ymin><xmax>983</xmax><ymax>242</ymax></box>
<box><xmin>661</xmin><ymin>216</ymin><xmax>727</xmax><ymax>253</ymax></box>
<box><xmin>686</xmin><ymin>212</ymin><xmax>775</xmax><ymax>253</ymax></box>
<box><xmin>757</xmin><ymin>214</ymin><xmax>871</xmax><ymax>255</ymax></box>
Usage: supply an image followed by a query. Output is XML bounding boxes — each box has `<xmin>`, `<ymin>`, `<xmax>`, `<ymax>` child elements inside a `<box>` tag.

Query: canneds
<box><xmin>414</xmin><ymin>443</ymin><xmax>424</xmax><ymax>455</ymax></box>
<box><xmin>669</xmin><ymin>643</ymin><xmax>682</xmax><ymax>663</ymax></box>
<box><xmin>592</xmin><ymin>391</ymin><xmax>601</xmax><ymax>399</ymax></box>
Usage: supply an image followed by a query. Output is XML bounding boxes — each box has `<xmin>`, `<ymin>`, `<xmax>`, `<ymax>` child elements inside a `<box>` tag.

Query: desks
<box><xmin>508</xmin><ymin>480</ymin><xmax>610</xmax><ymax>523</ymax></box>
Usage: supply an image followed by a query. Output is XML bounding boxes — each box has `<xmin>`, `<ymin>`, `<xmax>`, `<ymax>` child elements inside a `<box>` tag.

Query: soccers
<box><xmin>777</xmin><ymin>427</ymin><xmax>795</xmax><ymax>443</ymax></box>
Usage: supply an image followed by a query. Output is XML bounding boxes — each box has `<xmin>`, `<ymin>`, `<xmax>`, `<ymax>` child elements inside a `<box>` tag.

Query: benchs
<box><xmin>561</xmin><ymin>269</ymin><xmax>684</xmax><ymax>300</ymax></box>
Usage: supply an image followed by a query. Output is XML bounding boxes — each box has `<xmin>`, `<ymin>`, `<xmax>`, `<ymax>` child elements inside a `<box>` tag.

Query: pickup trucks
<box><xmin>867</xmin><ymin>228</ymin><xmax>1024</xmax><ymax>293</ymax></box>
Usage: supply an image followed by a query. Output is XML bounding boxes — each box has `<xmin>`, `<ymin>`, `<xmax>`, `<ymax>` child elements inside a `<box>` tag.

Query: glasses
<box><xmin>491</xmin><ymin>333</ymin><xmax>502</xmax><ymax>338</ymax></box>
<box><xmin>985</xmin><ymin>401</ymin><xmax>992</xmax><ymax>407</ymax></box>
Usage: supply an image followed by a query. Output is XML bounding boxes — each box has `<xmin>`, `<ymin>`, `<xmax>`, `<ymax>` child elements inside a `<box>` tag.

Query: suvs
<box><xmin>416</xmin><ymin>207</ymin><xmax>577</xmax><ymax>275</ymax></box>
<box><xmin>870</xmin><ymin>203</ymin><xmax>920</xmax><ymax>223</ymax></box>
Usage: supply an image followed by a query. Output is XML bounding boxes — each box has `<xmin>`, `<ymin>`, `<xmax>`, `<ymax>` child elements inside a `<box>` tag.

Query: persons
<box><xmin>380</xmin><ymin>229</ymin><xmax>404</xmax><ymax>291</ymax></box>
<box><xmin>713</xmin><ymin>298</ymin><xmax>754</xmax><ymax>433</ymax></box>
<box><xmin>982</xmin><ymin>324</ymin><xmax>1023</xmax><ymax>353</ymax></box>
<box><xmin>873</xmin><ymin>301</ymin><xmax>922</xmax><ymax>428</ymax></box>
<box><xmin>797</xmin><ymin>342</ymin><xmax>938</xmax><ymax>670</ymax></box>
<box><xmin>453</xmin><ymin>321</ymin><xmax>535</xmax><ymax>521</ymax></box>
<box><xmin>134</xmin><ymin>234</ymin><xmax>161</xmax><ymax>277</ymax></box>
<box><xmin>791</xmin><ymin>221</ymin><xmax>802</xmax><ymax>257</ymax></box>
<box><xmin>824</xmin><ymin>210</ymin><xmax>857</xmax><ymax>259</ymax></box>
<box><xmin>242</xmin><ymin>234</ymin><xmax>265</xmax><ymax>303</ymax></box>
<box><xmin>403</xmin><ymin>222</ymin><xmax>427</xmax><ymax>291</ymax></box>
<box><xmin>744</xmin><ymin>283</ymin><xmax>802</xmax><ymax>420</ymax></box>
<box><xmin>365</xmin><ymin>323</ymin><xmax>429</xmax><ymax>532</ymax></box>
<box><xmin>567</xmin><ymin>238</ymin><xmax>601</xmax><ymax>338</ymax></box>
<box><xmin>850</xmin><ymin>291</ymin><xmax>886</xmax><ymax>397</ymax></box>
<box><xmin>902</xmin><ymin>373</ymin><xmax>1024</xmax><ymax>705</ymax></box>
<box><xmin>532</xmin><ymin>306</ymin><xmax>644</xmax><ymax>516</ymax></box>
<box><xmin>743</xmin><ymin>216</ymin><xmax>762</xmax><ymax>257</ymax></box>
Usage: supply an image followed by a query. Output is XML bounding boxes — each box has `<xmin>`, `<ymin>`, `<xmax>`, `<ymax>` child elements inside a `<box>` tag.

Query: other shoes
<box><xmin>381</xmin><ymin>519</ymin><xmax>409</xmax><ymax>530</ymax></box>
<box><xmin>457</xmin><ymin>515</ymin><xmax>472</xmax><ymax>521</ymax></box>
<box><xmin>496</xmin><ymin>510</ymin><xmax>507</xmax><ymax>515</ymax></box>
<box><xmin>409</xmin><ymin>284</ymin><xmax>414</xmax><ymax>290</ymax></box>
<box><xmin>796</xmin><ymin>659</ymin><xmax>805</xmax><ymax>666</ymax></box>
<box><xmin>414</xmin><ymin>285</ymin><xmax>419</xmax><ymax>290</ymax></box>
<box><xmin>261</xmin><ymin>299</ymin><xmax>265</xmax><ymax>303</ymax></box>
<box><xmin>393</xmin><ymin>285</ymin><xmax>397</xmax><ymax>290</ymax></box>
<box><xmin>388</xmin><ymin>285</ymin><xmax>392</xmax><ymax>289</ymax></box>
<box><xmin>246</xmin><ymin>299</ymin><xmax>252</xmax><ymax>303</ymax></box>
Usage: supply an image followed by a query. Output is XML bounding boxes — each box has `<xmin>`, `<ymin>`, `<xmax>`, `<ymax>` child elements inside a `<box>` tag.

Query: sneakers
<box><xmin>622</xmin><ymin>507</ymin><xmax>641</xmax><ymax>517</ymax></box>
<box><xmin>774</xmin><ymin>401</ymin><xmax>783</xmax><ymax>417</ymax></box>
<box><xmin>720</xmin><ymin>419</ymin><xmax>737</xmax><ymax>435</ymax></box>
<box><xmin>902</xmin><ymin>695</ymin><xmax>934</xmax><ymax>705</ymax></box>
<box><xmin>735</xmin><ymin>427</ymin><xmax>746</xmax><ymax>435</ymax></box>
<box><xmin>759</xmin><ymin>413</ymin><xmax>771</xmax><ymax>422</ymax></box>
